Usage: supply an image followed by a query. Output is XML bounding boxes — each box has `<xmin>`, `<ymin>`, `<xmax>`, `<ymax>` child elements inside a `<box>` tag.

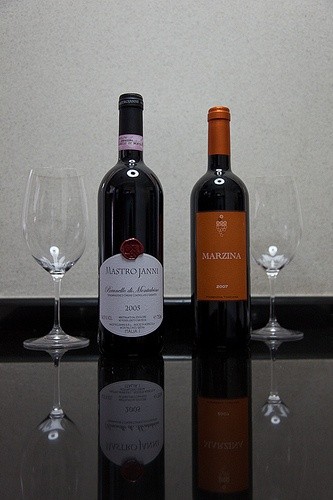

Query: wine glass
<box><xmin>252</xmin><ymin>175</ymin><xmax>305</xmax><ymax>338</ymax></box>
<box><xmin>23</xmin><ymin>167</ymin><xmax>90</xmax><ymax>349</ymax></box>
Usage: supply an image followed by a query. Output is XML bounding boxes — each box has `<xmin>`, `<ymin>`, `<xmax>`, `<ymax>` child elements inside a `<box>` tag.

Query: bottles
<box><xmin>98</xmin><ymin>93</ymin><xmax>163</xmax><ymax>357</ymax></box>
<box><xmin>190</xmin><ymin>106</ymin><xmax>251</xmax><ymax>359</ymax></box>
